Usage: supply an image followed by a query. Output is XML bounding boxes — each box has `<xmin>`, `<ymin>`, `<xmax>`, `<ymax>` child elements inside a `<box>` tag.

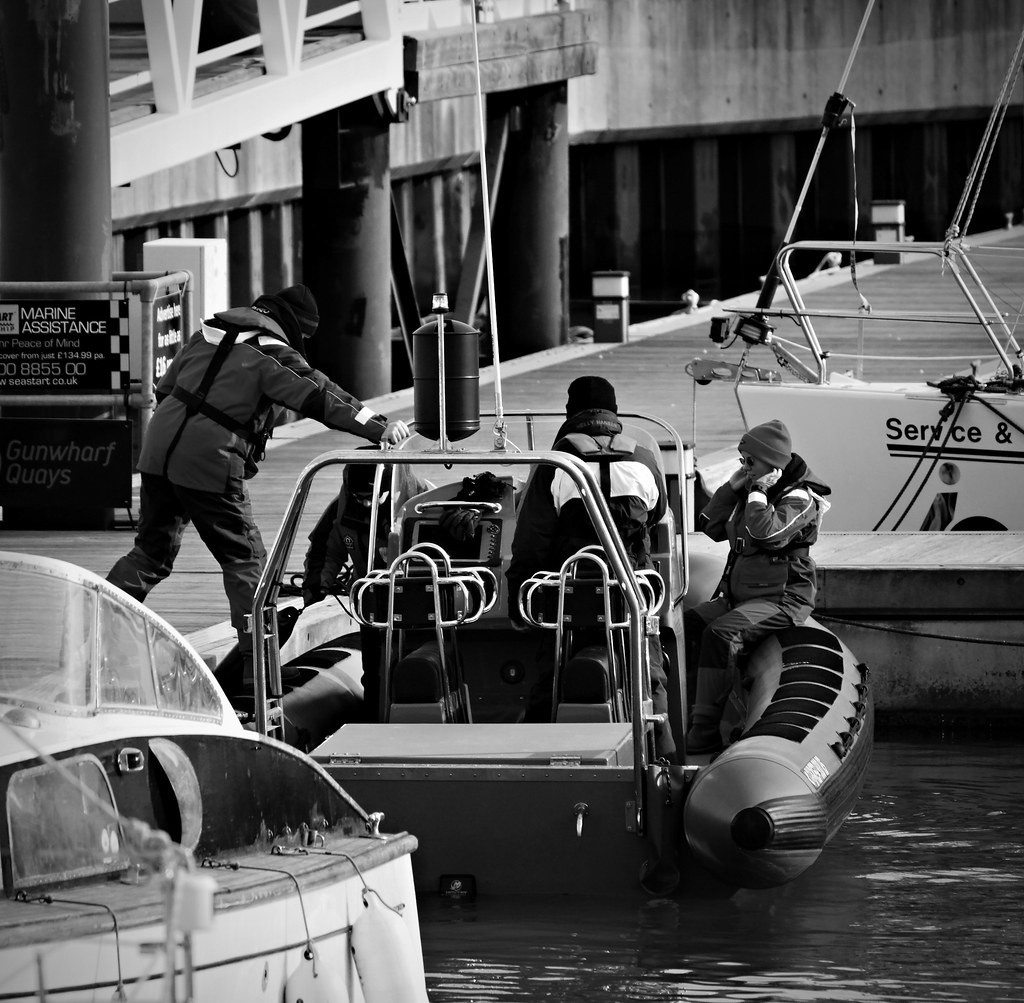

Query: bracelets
<box><xmin>750</xmin><ymin>485</ymin><xmax>768</xmax><ymax>495</ymax></box>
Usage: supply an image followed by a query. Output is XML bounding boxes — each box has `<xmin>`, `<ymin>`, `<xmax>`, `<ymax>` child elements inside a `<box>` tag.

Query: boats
<box><xmin>677</xmin><ymin>235</ymin><xmax>1024</xmax><ymax>715</ymax></box>
<box><xmin>0</xmin><ymin>552</ymin><xmax>434</xmax><ymax>1003</ymax></box>
<box><xmin>222</xmin><ymin>406</ymin><xmax>873</xmax><ymax>906</ymax></box>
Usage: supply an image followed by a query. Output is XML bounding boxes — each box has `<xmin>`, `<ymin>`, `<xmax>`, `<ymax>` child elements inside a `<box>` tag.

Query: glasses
<box><xmin>738</xmin><ymin>456</ymin><xmax>760</xmax><ymax>467</ymax></box>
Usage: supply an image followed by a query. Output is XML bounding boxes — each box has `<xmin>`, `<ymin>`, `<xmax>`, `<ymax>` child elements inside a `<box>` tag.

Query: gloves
<box><xmin>756</xmin><ymin>468</ymin><xmax>783</xmax><ymax>491</ymax></box>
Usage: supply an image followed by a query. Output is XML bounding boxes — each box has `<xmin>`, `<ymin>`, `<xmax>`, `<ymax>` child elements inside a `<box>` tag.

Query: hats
<box><xmin>336</xmin><ymin>444</ymin><xmax>418</xmax><ymax>525</ymax></box>
<box><xmin>566</xmin><ymin>376</ymin><xmax>618</xmax><ymax>418</ymax></box>
<box><xmin>275</xmin><ymin>283</ymin><xmax>320</xmax><ymax>336</ymax></box>
<box><xmin>738</xmin><ymin>419</ymin><xmax>792</xmax><ymax>470</ymax></box>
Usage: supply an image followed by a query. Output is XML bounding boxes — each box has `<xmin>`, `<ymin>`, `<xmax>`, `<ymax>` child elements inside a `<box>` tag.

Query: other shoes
<box><xmin>687</xmin><ymin>722</ymin><xmax>723</xmax><ymax>752</ymax></box>
<box><xmin>243</xmin><ymin>656</ymin><xmax>300</xmax><ymax>685</ymax></box>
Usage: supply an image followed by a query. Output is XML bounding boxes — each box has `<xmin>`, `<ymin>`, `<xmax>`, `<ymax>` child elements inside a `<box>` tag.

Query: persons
<box><xmin>504</xmin><ymin>376</ymin><xmax>677</xmax><ymax>761</ymax></box>
<box><xmin>107</xmin><ymin>283</ymin><xmax>411</xmax><ymax>684</ymax></box>
<box><xmin>301</xmin><ymin>445</ymin><xmax>428</xmax><ymax>604</ymax></box>
<box><xmin>663</xmin><ymin>419</ymin><xmax>832</xmax><ymax>754</ymax></box>
